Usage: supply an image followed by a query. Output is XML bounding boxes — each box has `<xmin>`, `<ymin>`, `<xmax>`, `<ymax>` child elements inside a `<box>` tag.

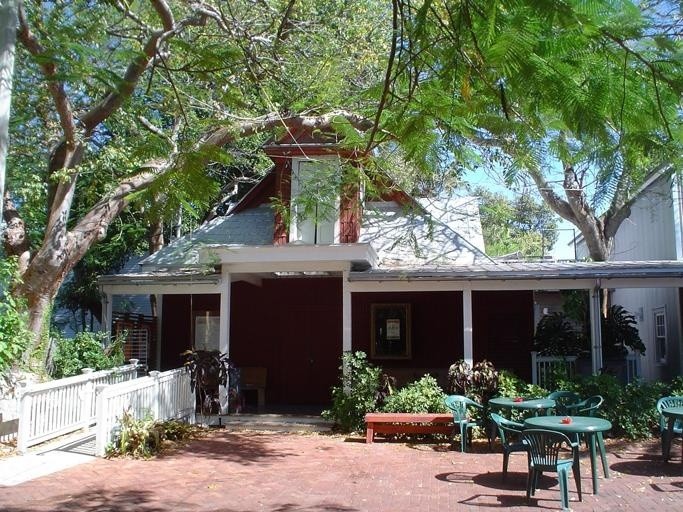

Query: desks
<box><xmin>662</xmin><ymin>407</ymin><xmax>683</xmax><ymax>462</ymax></box>
<box><xmin>524</xmin><ymin>416</ymin><xmax>612</xmax><ymax>495</ymax></box>
<box><xmin>488</xmin><ymin>397</ymin><xmax>555</xmax><ymax>451</ymax></box>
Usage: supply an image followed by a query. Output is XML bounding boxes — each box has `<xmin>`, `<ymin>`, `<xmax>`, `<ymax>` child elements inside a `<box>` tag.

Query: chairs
<box><xmin>523</xmin><ymin>429</ymin><xmax>583</xmax><ymax>511</ymax></box>
<box><xmin>547</xmin><ymin>391</ymin><xmax>581</xmax><ymax>416</ymax></box>
<box><xmin>657</xmin><ymin>397</ymin><xmax>683</xmax><ymax>455</ymax></box>
<box><xmin>565</xmin><ymin>395</ymin><xmax>604</xmax><ymax>448</ymax></box>
<box><xmin>444</xmin><ymin>395</ymin><xmax>490</xmax><ymax>452</ymax></box>
<box><xmin>490</xmin><ymin>413</ymin><xmax>531</xmax><ymax>476</ymax></box>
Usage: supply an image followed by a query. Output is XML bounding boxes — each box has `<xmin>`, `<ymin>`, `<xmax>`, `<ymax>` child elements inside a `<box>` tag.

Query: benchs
<box><xmin>364</xmin><ymin>412</ymin><xmax>472</xmax><ymax>447</ymax></box>
